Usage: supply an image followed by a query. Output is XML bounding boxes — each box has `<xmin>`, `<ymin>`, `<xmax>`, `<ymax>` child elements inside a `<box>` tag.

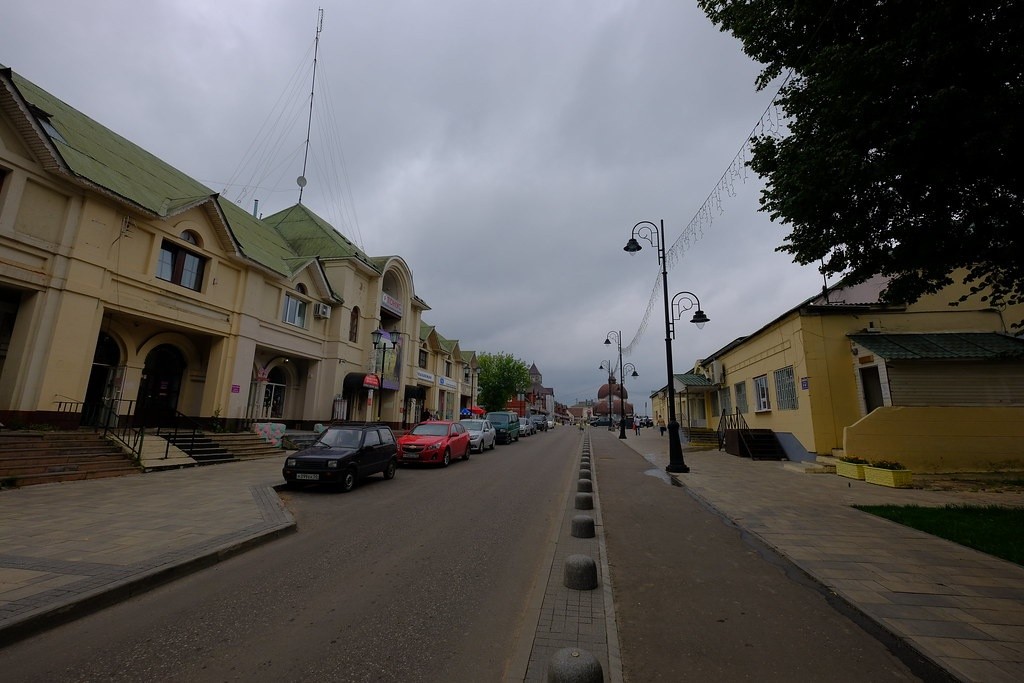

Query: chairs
<box><xmin>339</xmin><ymin>433</ymin><xmax>353</xmax><ymax>446</ymax></box>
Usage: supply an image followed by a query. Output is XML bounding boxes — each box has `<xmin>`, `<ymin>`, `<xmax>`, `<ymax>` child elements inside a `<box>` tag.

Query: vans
<box><xmin>486</xmin><ymin>411</ymin><xmax>519</xmax><ymax>445</ymax></box>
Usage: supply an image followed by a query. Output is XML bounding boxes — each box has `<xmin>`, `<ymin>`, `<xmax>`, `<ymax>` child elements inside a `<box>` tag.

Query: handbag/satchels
<box><xmin>664</xmin><ymin>426</ymin><xmax>666</xmax><ymax>431</ymax></box>
<box><xmin>437</xmin><ymin>414</ymin><xmax>440</xmax><ymax>419</ymax></box>
<box><xmin>633</xmin><ymin>423</ymin><xmax>636</xmax><ymax>430</ymax></box>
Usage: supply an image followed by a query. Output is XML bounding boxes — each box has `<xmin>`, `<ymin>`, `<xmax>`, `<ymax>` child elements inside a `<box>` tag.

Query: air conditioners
<box><xmin>709</xmin><ymin>360</ymin><xmax>725</xmax><ymax>385</ymax></box>
<box><xmin>313</xmin><ymin>302</ymin><xmax>331</xmax><ymax>319</ymax></box>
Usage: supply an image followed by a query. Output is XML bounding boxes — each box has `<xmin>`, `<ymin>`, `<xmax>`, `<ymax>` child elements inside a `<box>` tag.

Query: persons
<box><xmin>634</xmin><ymin>416</ymin><xmax>640</xmax><ymax>436</ymax></box>
<box><xmin>434</xmin><ymin>411</ymin><xmax>439</xmax><ymax>421</ymax></box>
<box><xmin>579</xmin><ymin>416</ymin><xmax>584</xmax><ymax>430</ymax></box>
<box><xmin>423</xmin><ymin>408</ymin><xmax>430</xmax><ymax>421</ymax></box>
<box><xmin>658</xmin><ymin>414</ymin><xmax>665</xmax><ymax>436</ymax></box>
<box><xmin>646</xmin><ymin>416</ymin><xmax>649</xmax><ymax>428</ymax></box>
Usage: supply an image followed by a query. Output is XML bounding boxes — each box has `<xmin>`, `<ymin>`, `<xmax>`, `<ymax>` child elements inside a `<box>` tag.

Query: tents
<box><xmin>468</xmin><ymin>406</ymin><xmax>486</xmax><ymax>414</ymax></box>
<box><xmin>459</xmin><ymin>407</ymin><xmax>471</xmax><ymax>416</ymax></box>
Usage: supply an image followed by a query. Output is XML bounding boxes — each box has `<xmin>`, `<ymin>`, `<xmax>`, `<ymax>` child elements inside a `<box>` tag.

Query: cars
<box><xmin>458</xmin><ymin>418</ymin><xmax>496</xmax><ymax>455</ymax></box>
<box><xmin>590</xmin><ymin>417</ymin><xmax>616</xmax><ymax>427</ymax></box>
<box><xmin>396</xmin><ymin>421</ymin><xmax>471</xmax><ymax>468</ymax></box>
<box><xmin>518</xmin><ymin>418</ymin><xmax>536</xmax><ymax>437</ymax></box>
<box><xmin>547</xmin><ymin>417</ymin><xmax>555</xmax><ymax>429</ymax></box>
<box><xmin>640</xmin><ymin>418</ymin><xmax>653</xmax><ymax>428</ymax></box>
<box><xmin>282</xmin><ymin>425</ymin><xmax>398</xmax><ymax>492</ymax></box>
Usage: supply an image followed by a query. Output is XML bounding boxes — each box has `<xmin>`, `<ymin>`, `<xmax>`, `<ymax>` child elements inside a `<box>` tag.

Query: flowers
<box><xmin>838</xmin><ymin>453</ymin><xmax>870</xmax><ymax>464</ymax></box>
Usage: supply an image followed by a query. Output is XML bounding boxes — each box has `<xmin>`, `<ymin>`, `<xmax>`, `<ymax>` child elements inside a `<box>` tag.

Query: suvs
<box><xmin>529</xmin><ymin>414</ymin><xmax>548</xmax><ymax>432</ymax></box>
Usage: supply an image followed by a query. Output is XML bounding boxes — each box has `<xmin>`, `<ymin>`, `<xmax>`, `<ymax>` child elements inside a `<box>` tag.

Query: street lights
<box><xmin>621</xmin><ymin>218</ymin><xmax>712</xmax><ymax>474</ymax></box>
<box><xmin>604</xmin><ymin>330</ymin><xmax>640</xmax><ymax>439</ymax></box>
<box><xmin>599</xmin><ymin>360</ymin><xmax>619</xmax><ymax>428</ymax></box>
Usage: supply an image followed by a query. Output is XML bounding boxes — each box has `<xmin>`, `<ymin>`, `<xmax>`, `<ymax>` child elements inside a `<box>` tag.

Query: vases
<box><xmin>836</xmin><ymin>460</ymin><xmax>871</xmax><ymax>479</ymax></box>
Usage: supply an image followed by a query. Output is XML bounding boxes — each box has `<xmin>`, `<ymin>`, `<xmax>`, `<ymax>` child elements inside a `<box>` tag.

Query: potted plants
<box><xmin>863</xmin><ymin>461</ymin><xmax>913</xmax><ymax>488</ymax></box>
